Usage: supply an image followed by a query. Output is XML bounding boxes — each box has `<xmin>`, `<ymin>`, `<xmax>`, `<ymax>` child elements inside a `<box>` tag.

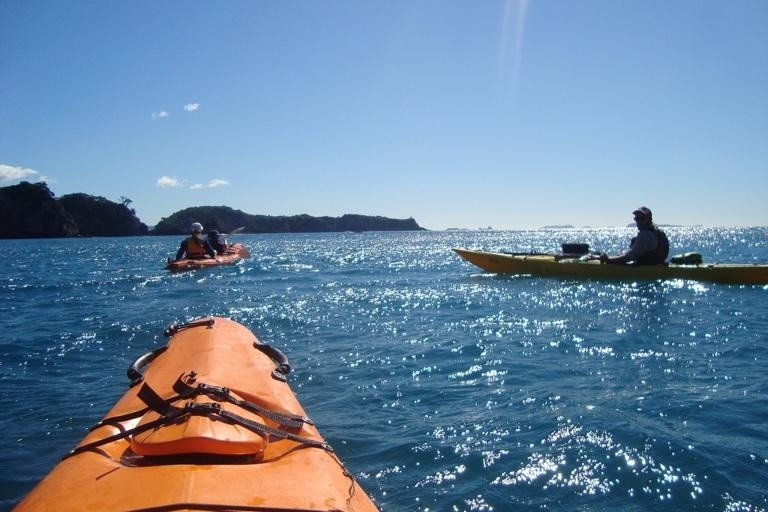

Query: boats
<box><xmin>449</xmin><ymin>250</ymin><xmax>768</xmax><ymax>286</ymax></box>
<box><xmin>14</xmin><ymin>316</ymin><xmax>383</xmax><ymax>512</ymax></box>
<box><xmin>169</xmin><ymin>241</ymin><xmax>246</xmax><ymax>270</ymax></box>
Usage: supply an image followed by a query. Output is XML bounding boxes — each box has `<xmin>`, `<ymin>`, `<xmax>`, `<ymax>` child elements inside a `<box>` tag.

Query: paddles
<box><xmin>219</xmin><ymin>227</ymin><xmax>245</xmax><ymax>241</ymax></box>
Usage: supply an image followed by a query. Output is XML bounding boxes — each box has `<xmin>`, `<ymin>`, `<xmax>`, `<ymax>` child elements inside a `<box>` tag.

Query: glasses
<box><xmin>634</xmin><ymin>217</ymin><xmax>645</xmax><ymax>221</ymax></box>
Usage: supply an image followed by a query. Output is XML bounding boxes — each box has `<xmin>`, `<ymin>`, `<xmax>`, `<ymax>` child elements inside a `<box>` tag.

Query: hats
<box><xmin>191</xmin><ymin>222</ymin><xmax>203</xmax><ymax>231</ymax></box>
<box><xmin>632</xmin><ymin>207</ymin><xmax>652</xmax><ymax>217</ymax></box>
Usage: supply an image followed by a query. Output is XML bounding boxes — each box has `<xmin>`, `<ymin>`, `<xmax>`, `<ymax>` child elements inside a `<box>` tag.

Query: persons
<box><xmin>601</xmin><ymin>205</ymin><xmax>671</xmax><ymax>266</ymax></box>
<box><xmin>202</xmin><ymin>230</ymin><xmax>225</xmax><ymax>256</ymax></box>
<box><xmin>174</xmin><ymin>223</ymin><xmax>218</xmax><ymax>262</ymax></box>
